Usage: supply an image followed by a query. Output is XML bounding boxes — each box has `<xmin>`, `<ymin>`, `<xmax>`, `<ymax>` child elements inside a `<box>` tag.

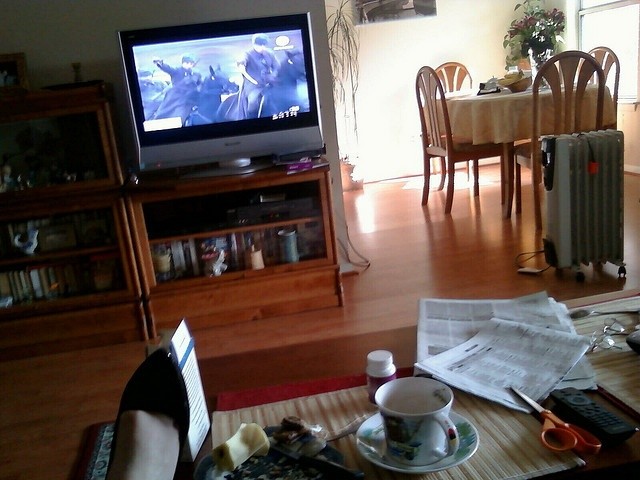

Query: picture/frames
<box><xmin>1</xmin><ymin>51</ymin><xmax>29</xmax><ymax>93</ymax></box>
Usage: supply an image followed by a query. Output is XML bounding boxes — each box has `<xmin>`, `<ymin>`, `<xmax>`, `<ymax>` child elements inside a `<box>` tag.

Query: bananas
<box><xmin>498</xmin><ymin>70</ymin><xmax>526</xmax><ymax>86</ymax></box>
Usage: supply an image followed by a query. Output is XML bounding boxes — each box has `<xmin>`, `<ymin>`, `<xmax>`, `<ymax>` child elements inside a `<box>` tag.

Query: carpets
<box><xmin>67</xmin><ymin>420</ymin><xmax>116</xmax><ymax>480</ymax></box>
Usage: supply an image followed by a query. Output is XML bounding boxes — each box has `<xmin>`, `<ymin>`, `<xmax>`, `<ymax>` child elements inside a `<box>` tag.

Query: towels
<box><xmin>210</xmin><ymin>376</ymin><xmax>586</xmax><ymax>480</ymax></box>
<box><xmin>555</xmin><ymin>287</ymin><xmax>640</xmax><ymax>422</ymax></box>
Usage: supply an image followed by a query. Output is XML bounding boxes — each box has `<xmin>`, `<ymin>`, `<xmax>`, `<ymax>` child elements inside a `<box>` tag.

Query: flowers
<box><xmin>503</xmin><ymin>0</ymin><xmax>568</xmax><ymax>71</ymax></box>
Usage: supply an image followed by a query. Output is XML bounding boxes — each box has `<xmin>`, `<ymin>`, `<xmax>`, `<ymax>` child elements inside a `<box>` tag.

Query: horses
<box><xmin>146</xmin><ymin>61</ymin><xmax>240</xmax><ymax>127</ymax></box>
<box><xmin>215</xmin><ymin>50</ymin><xmax>311</xmax><ymax>122</ymax></box>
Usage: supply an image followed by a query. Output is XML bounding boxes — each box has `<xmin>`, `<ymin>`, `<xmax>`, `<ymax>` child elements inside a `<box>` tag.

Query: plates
<box><xmin>355</xmin><ymin>412</ymin><xmax>479</xmax><ymax>474</ymax></box>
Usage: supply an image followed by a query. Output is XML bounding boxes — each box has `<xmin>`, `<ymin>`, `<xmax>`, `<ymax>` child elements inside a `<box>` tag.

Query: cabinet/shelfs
<box><xmin>0</xmin><ymin>194</ymin><xmax>143</xmax><ymax>319</ymax></box>
<box><xmin>123</xmin><ymin>155</ymin><xmax>343</xmax><ymax>339</ymax></box>
<box><xmin>1</xmin><ymin>80</ymin><xmax>148</xmax><ymax>204</ymax></box>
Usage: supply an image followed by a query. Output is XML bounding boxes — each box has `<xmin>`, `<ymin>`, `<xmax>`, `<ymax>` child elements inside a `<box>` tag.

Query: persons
<box><xmin>157</xmin><ymin>55</ymin><xmax>195</xmax><ymax>85</ymax></box>
<box><xmin>243</xmin><ymin>38</ymin><xmax>279</xmax><ymax>118</ymax></box>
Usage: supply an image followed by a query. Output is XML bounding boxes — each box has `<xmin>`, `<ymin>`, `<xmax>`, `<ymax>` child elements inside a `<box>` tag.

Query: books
<box><xmin>151</xmin><ymin>221</ymin><xmax>325</xmax><ymax>285</ymax></box>
<box><xmin>274</xmin><ymin>153</ymin><xmax>330</xmax><ymax>176</ymax></box>
<box><xmin>1</xmin><ymin>252</ymin><xmax>123</xmax><ymax>305</ymax></box>
<box><xmin>0</xmin><ymin>211</ymin><xmax>113</xmax><ymax>254</ymax></box>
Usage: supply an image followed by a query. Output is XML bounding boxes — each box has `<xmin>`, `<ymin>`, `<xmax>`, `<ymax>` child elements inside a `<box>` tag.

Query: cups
<box><xmin>277</xmin><ymin>230</ymin><xmax>306</xmax><ymax>262</ymax></box>
<box><xmin>374</xmin><ymin>377</ymin><xmax>459</xmax><ymax>466</ymax></box>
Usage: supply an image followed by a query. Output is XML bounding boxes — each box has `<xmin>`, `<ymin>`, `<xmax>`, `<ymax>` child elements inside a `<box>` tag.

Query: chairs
<box><xmin>434</xmin><ymin>61</ymin><xmax>474</xmax><ymax>191</ymax></box>
<box><xmin>579</xmin><ymin>46</ymin><xmax>620</xmax><ymax>117</ymax></box>
<box><xmin>415</xmin><ymin>65</ymin><xmax>504</xmax><ymax>214</ymax></box>
<box><xmin>512</xmin><ymin>50</ymin><xmax>606</xmax><ymax>235</ymax></box>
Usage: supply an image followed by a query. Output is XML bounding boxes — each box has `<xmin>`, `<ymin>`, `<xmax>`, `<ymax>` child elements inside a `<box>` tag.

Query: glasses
<box><xmin>588</xmin><ymin>317</ymin><xmax>629</xmax><ymax>353</ymax></box>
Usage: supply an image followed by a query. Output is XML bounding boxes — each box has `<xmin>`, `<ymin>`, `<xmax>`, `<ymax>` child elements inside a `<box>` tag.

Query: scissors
<box><xmin>510</xmin><ymin>385</ymin><xmax>603</xmax><ymax>455</ymax></box>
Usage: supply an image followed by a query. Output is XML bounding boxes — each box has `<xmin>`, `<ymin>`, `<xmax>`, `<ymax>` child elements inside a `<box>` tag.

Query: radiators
<box><xmin>538</xmin><ymin>130</ymin><xmax>627</xmax><ymax>284</ymax></box>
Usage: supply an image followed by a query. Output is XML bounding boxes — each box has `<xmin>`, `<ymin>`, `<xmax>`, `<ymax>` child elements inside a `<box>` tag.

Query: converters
<box><xmin>517</xmin><ymin>265</ymin><xmax>542</xmax><ymax>275</ymax></box>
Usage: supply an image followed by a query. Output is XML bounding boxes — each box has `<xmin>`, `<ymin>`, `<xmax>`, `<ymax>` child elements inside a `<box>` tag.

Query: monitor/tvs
<box><xmin>116</xmin><ymin>11</ymin><xmax>325</xmax><ymax>179</ymax></box>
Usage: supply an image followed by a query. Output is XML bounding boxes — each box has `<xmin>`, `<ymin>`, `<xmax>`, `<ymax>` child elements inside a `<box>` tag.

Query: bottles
<box><xmin>366</xmin><ymin>350</ymin><xmax>397</xmax><ymax>406</ymax></box>
<box><xmin>154</xmin><ymin>244</ymin><xmax>170</xmax><ymax>280</ymax></box>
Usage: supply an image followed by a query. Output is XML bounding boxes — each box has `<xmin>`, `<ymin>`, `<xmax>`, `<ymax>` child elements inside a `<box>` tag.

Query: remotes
<box><xmin>551</xmin><ymin>386</ymin><xmax>636</xmax><ymax>438</ymax></box>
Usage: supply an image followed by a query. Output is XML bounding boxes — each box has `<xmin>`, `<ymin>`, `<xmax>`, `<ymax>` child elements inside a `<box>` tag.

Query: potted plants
<box><xmin>324</xmin><ymin>0</ymin><xmax>365</xmax><ymax>193</ymax></box>
